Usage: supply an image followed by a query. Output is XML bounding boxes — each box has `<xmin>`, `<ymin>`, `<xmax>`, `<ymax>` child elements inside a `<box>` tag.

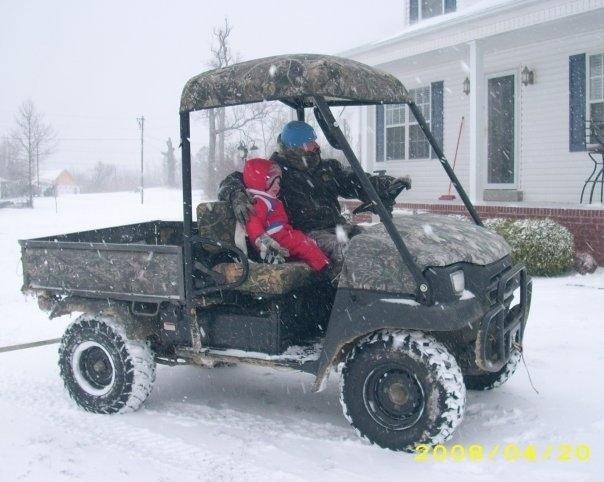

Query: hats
<box><xmin>265</xmin><ymin>163</ymin><xmax>283</xmax><ymax>191</ymax></box>
<box><xmin>281</xmin><ymin>120</ymin><xmax>318</xmax><ymax>148</ymax></box>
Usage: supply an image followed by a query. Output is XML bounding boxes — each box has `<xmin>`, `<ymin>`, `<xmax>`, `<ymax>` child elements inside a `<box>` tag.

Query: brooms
<box><xmin>438</xmin><ymin>116</ymin><xmax>464</xmax><ymax>200</ymax></box>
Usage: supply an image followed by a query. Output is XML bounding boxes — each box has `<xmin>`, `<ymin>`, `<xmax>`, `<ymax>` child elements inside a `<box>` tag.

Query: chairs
<box><xmin>580</xmin><ymin>117</ymin><xmax>603</xmax><ymax>203</ymax></box>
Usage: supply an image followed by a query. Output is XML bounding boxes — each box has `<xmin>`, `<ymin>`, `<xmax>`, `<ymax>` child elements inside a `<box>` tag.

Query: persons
<box><xmin>218</xmin><ymin>120</ymin><xmax>411</xmax><ymax>330</ymax></box>
<box><xmin>243</xmin><ymin>158</ymin><xmax>338</xmax><ymax>290</ymax></box>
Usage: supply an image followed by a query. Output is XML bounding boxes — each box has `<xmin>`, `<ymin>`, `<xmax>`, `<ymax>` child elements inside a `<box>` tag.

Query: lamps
<box><xmin>463</xmin><ymin>76</ymin><xmax>471</xmax><ymax>95</ymax></box>
<box><xmin>520</xmin><ymin>65</ymin><xmax>534</xmax><ymax>87</ymax></box>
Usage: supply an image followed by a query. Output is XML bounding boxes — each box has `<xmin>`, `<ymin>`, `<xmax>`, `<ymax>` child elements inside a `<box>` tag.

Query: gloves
<box><xmin>388</xmin><ymin>175</ymin><xmax>412</xmax><ymax>195</ymax></box>
<box><xmin>229</xmin><ymin>189</ymin><xmax>257</xmax><ymax>224</ymax></box>
<box><xmin>255</xmin><ymin>233</ymin><xmax>290</xmax><ymax>265</ymax></box>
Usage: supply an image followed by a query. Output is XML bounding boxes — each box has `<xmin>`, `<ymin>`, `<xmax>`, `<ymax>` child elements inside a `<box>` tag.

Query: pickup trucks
<box><xmin>18</xmin><ymin>54</ymin><xmax>532</xmax><ymax>453</ymax></box>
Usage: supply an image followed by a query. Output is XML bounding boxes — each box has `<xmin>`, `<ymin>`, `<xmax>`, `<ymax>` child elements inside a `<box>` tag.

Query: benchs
<box><xmin>196</xmin><ymin>201</ymin><xmax>312</xmax><ymax>299</ymax></box>
